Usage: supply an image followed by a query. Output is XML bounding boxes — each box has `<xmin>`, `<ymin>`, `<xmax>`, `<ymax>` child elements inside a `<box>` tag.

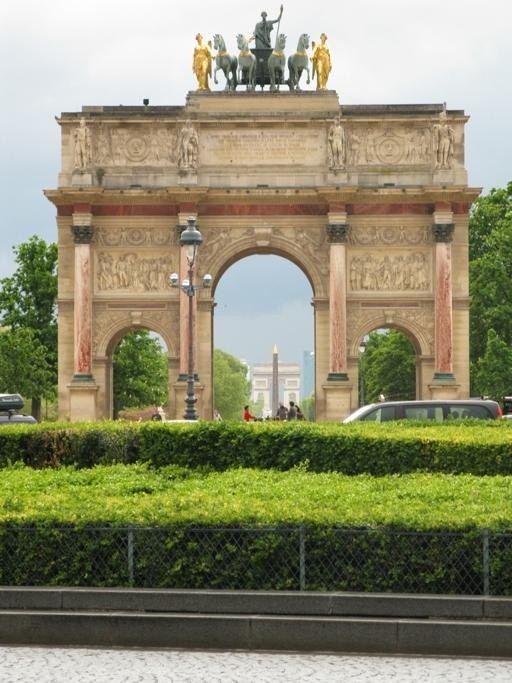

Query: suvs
<box><xmin>0</xmin><ymin>394</ymin><xmax>39</xmax><ymax>425</ymax></box>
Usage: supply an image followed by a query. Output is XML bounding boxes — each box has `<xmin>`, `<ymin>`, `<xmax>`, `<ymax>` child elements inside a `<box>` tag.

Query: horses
<box><xmin>213</xmin><ymin>33</ymin><xmax>238</xmax><ymax>91</ymax></box>
<box><xmin>268</xmin><ymin>33</ymin><xmax>287</xmax><ymax>93</ymax></box>
<box><xmin>236</xmin><ymin>33</ymin><xmax>257</xmax><ymax>92</ymax></box>
<box><xmin>288</xmin><ymin>33</ymin><xmax>311</xmax><ymax>93</ymax></box>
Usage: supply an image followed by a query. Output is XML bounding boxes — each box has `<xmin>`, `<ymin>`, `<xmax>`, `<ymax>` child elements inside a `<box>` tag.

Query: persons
<box><xmin>327</xmin><ymin>112</ymin><xmax>454</xmax><ymax>169</ymax></box>
<box><xmin>75</xmin><ymin>119</ymin><xmax>199</xmax><ymax>168</ymax></box>
<box><xmin>213</xmin><ymin>410</ymin><xmax>221</xmax><ymax>421</ymax></box>
<box><xmin>151</xmin><ymin>407</ymin><xmax>162</xmax><ymax>421</ymax></box>
<box><xmin>192</xmin><ymin>33</ymin><xmax>211</xmax><ymax>90</ymax></box>
<box><xmin>243</xmin><ymin>405</ymin><xmax>255</xmax><ymax>421</ymax></box>
<box><xmin>453</xmin><ymin>409</ymin><xmax>477</xmax><ymax>418</ymax></box>
<box><xmin>347</xmin><ymin>252</ymin><xmax>432</xmax><ymax>290</ymax></box>
<box><xmin>101</xmin><ymin>250</ymin><xmax>175</xmax><ymax>293</ymax></box>
<box><xmin>276</xmin><ymin>401</ymin><xmax>303</xmax><ymax>419</ymax></box>
<box><xmin>255</xmin><ymin>4</ymin><xmax>283</xmax><ymax>49</ymax></box>
<box><xmin>311</xmin><ymin>33</ymin><xmax>332</xmax><ymax>91</ymax></box>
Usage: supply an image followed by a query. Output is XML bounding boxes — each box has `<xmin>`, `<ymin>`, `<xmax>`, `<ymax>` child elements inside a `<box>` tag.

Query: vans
<box><xmin>340</xmin><ymin>399</ymin><xmax>502</xmax><ymax>424</ymax></box>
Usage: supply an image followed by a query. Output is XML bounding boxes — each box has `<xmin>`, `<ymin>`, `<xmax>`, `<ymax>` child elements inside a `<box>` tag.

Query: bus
<box><xmin>496</xmin><ymin>395</ymin><xmax>512</xmax><ymax>420</ymax></box>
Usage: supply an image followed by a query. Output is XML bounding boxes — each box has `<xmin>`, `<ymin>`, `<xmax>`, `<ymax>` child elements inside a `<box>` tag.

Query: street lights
<box><xmin>169</xmin><ymin>214</ymin><xmax>212</xmax><ymax>419</ymax></box>
<box><xmin>359</xmin><ymin>338</ymin><xmax>367</xmax><ymax>407</ymax></box>
